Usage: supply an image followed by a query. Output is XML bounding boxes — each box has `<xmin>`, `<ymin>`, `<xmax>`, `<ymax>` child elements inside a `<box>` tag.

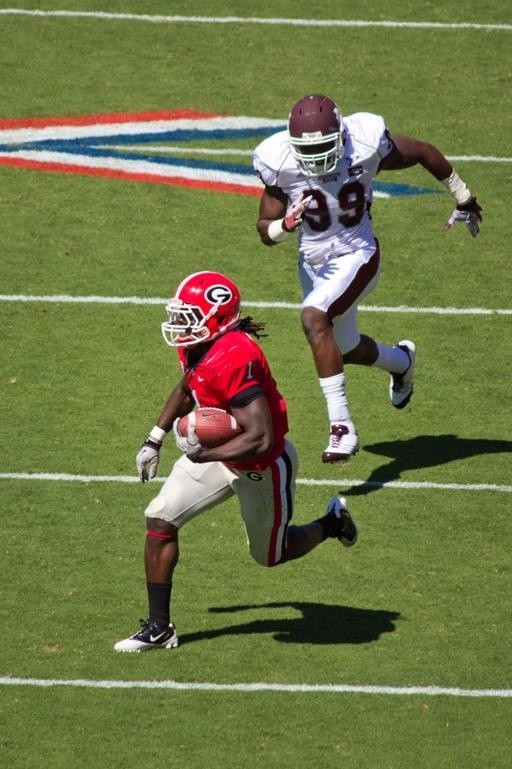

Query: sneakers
<box><xmin>389</xmin><ymin>340</ymin><xmax>416</xmax><ymax>408</ymax></box>
<box><xmin>327</xmin><ymin>496</ymin><xmax>356</xmax><ymax>546</ymax></box>
<box><xmin>322</xmin><ymin>422</ymin><xmax>359</xmax><ymax>464</ymax></box>
<box><xmin>114</xmin><ymin>619</ymin><xmax>178</xmax><ymax>652</ymax></box>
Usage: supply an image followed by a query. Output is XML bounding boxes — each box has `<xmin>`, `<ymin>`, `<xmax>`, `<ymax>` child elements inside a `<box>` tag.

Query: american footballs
<box><xmin>179</xmin><ymin>407</ymin><xmax>245</xmax><ymax>447</ymax></box>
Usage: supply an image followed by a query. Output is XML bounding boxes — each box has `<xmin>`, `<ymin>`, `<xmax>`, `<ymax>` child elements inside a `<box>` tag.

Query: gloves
<box><xmin>136</xmin><ymin>435</ymin><xmax>162</xmax><ymax>484</ymax></box>
<box><xmin>282</xmin><ymin>193</ymin><xmax>312</xmax><ymax>232</ymax></box>
<box><xmin>173</xmin><ymin>411</ymin><xmax>203</xmax><ymax>463</ymax></box>
<box><xmin>445</xmin><ymin>197</ymin><xmax>482</xmax><ymax>237</ymax></box>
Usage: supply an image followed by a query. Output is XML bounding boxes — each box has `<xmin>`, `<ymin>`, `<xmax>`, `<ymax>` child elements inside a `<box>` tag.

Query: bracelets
<box><xmin>148</xmin><ymin>424</ymin><xmax>167</xmax><ymax>442</ymax></box>
<box><xmin>266</xmin><ymin>218</ymin><xmax>291</xmax><ymax>244</ymax></box>
<box><xmin>438</xmin><ymin>167</ymin><xmax>473</xmax><ymax>205</ymax></box>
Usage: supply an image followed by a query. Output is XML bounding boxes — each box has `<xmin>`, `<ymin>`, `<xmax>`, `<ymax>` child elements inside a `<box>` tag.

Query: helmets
<box><xmin>287</xmin><ymin>94</ymin><xmax>344</xmax><ymax>177</ymax></box>
<box><xmin>161</xmin><ymin>271</ymin><xmax>240</xmax><ymax>346</ymax></box>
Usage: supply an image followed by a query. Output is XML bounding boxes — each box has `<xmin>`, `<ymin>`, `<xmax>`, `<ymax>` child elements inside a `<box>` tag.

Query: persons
<box><xmin>111</xmin><ymin>268</ymin><xmax>360</xmax><ymax>656</ymax></box>
<box><xmin>246</xmin><ymin>93</ymin><xmax>484</xmax><ymax>466</ymax></box>
